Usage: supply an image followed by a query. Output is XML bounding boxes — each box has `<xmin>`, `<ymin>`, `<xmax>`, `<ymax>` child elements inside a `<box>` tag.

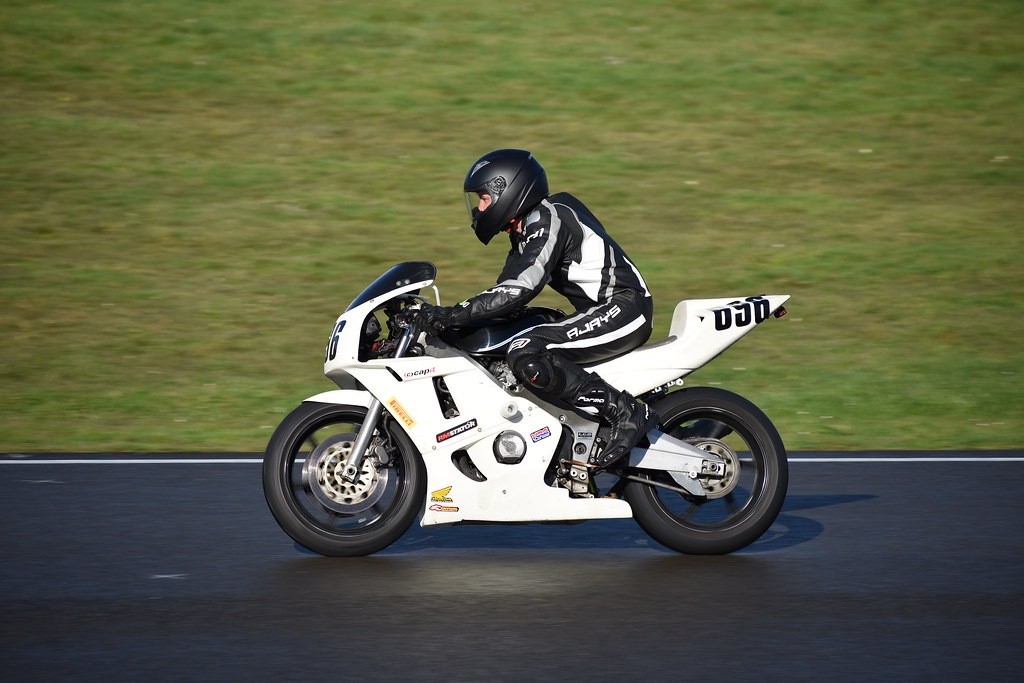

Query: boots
<box><xmin>569</xmin><ymin>371</ymin><xmax>660</xmax><ymax>467</ymax></box>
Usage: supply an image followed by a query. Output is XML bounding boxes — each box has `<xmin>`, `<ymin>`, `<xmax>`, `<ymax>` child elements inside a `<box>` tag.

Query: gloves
<box><xmin>416</xmin><ymin>304</ymin><xmax>471</xmax><ymax>336</ymax></box>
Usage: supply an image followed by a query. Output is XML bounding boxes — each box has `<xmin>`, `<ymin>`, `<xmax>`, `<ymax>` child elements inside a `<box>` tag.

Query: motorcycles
<box><xmin>259</xmin><ymin>258</ymin><xmax>793</xmax><ymax>556</ymax></box>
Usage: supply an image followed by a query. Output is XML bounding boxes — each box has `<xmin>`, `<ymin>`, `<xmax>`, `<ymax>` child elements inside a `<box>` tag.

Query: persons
<box><xmin>415</xmin><ymin>148</ymin><xmax>663</xmax><ymax>468</ymax></box>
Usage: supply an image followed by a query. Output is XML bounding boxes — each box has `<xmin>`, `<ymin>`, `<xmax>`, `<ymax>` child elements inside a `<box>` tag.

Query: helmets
<box><xmin>464</xmin><ymin>149</ymin><xmax>548</xmax><ymax>245</ymax></box>
